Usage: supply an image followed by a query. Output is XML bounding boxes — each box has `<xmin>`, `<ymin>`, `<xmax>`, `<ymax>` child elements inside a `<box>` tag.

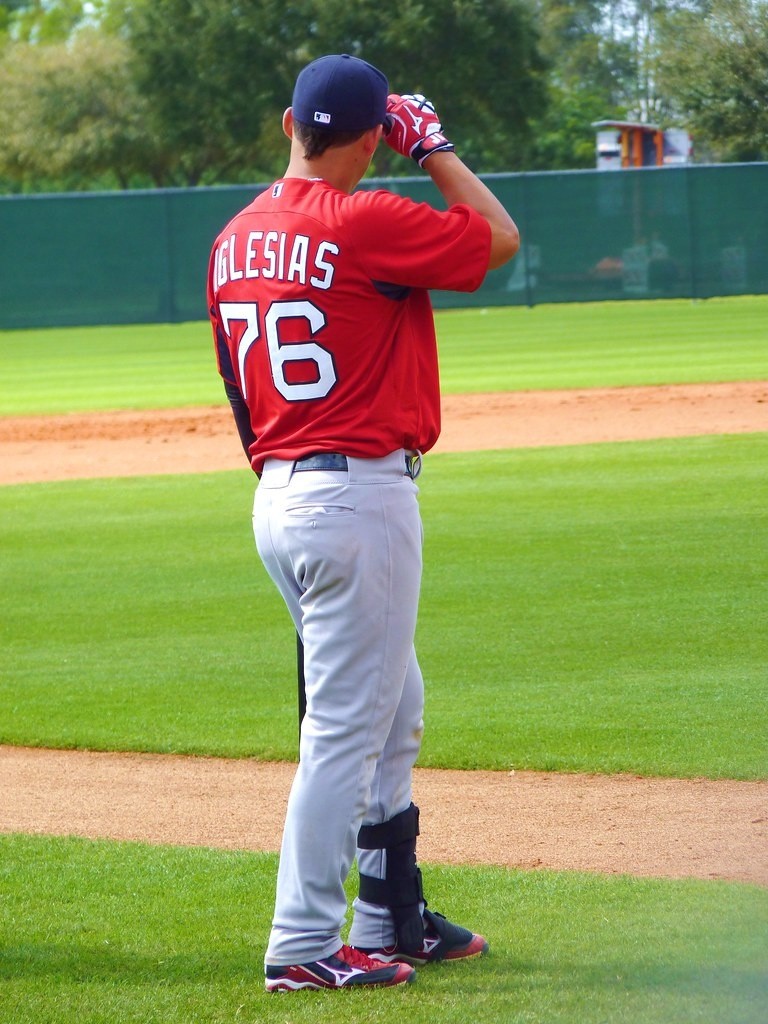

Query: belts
<box><xmin>292</xmin><ymin>453</ymin><xmax>413</xmax><ymax>477</ymax></box>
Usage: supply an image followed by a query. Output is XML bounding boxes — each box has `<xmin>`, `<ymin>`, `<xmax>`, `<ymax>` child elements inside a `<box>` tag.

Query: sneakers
<box><xmin>347</xmin><ymin>899</ymin><xmax>489</xmax><ymax>966</ymax></box>
<box><xmin>265</xmin><ymin>927</ymin><xmax>417</xmax><ymax>993</ymax></box>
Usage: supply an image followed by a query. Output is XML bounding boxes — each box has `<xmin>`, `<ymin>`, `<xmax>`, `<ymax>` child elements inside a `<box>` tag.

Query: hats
<box><xmin>291</xmin><ymin>54</ymin><xmax>392</xmax><ymax>131</ymax></box>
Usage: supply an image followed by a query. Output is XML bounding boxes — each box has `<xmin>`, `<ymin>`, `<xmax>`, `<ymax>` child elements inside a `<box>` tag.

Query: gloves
<box><xmin>380</xmin><ymin>94</ymin><xmax>455</xmax><ymax>169</ymax></box>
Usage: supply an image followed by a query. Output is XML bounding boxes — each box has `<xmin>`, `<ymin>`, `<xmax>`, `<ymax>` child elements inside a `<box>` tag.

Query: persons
<box><xmin>206</xmin><ymin>54</ymin><xmax>519</xmax><ymax>994</ymax></box>
